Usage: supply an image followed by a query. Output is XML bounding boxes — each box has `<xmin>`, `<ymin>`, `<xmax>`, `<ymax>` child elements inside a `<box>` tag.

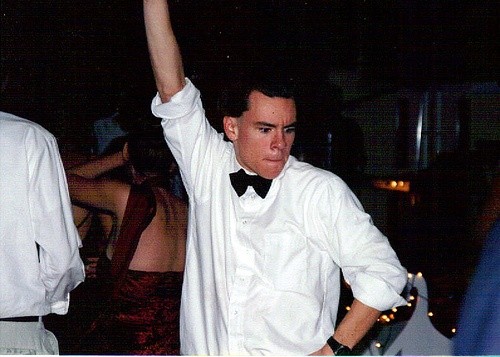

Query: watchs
<box><xmin>325</xmin><ymin>337</ymin><xmax>352</xmax><ymax>356</ymax></box>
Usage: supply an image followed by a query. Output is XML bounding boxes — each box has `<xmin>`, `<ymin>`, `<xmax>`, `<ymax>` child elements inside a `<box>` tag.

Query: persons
<box><xmin>60</xmin><ymin>136</ymin><xmax>500</xmax><ymax>355</ymax></box>
<box><xmin>144</xmin><ymin>0</ymin><xmax>409</xmax><ymax>355</ymax></box>
<box><xmin>0</xmin><ymin>110</ymin><xmax>88</xmax><ymax>355</ymax></box>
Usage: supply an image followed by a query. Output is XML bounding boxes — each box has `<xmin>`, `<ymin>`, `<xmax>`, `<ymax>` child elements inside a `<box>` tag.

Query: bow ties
<box><xmin>229</xmin><ymin>168</ymin><xmax>274</xmax><ymax>199</ymax></box>
